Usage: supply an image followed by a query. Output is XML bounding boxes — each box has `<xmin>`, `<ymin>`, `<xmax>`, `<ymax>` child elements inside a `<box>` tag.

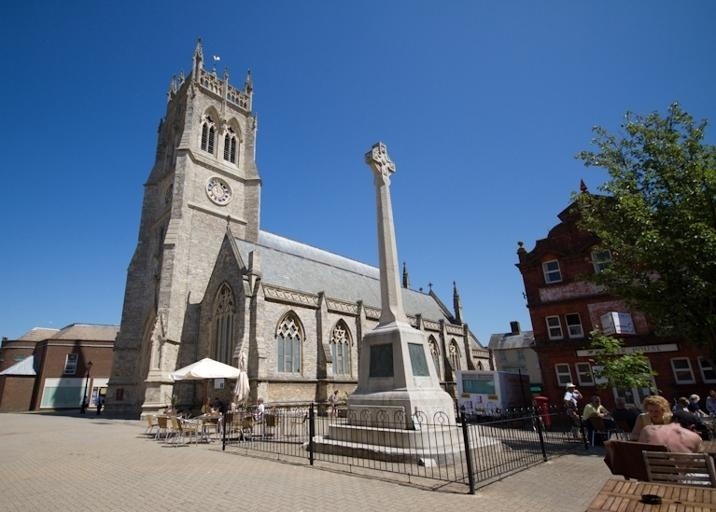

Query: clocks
<box><xmin>205</xmin><ymin>176</ymin><xmax>234</xmax><ymax>207</ymax></box>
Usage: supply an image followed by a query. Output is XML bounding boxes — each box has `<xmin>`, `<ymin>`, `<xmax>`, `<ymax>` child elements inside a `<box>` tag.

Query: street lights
<box><xmin>80</xmin><ymin>360</ymin><xmax>93</xmax><ymax>414</ymax></box>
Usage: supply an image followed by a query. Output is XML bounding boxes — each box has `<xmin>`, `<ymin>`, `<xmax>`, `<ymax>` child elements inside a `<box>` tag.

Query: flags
<box><xmin>213</xmin><ymin>54</ymin><xmax>221</xmax><ymax>61</ymax></box>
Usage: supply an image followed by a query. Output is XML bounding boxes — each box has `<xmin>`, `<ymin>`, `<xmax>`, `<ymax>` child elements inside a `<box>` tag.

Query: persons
<box><xmin>327</xmin><ymin>390</ymin><xmax>341</xmax><ymax>418</ymax></box>
<box><xmin>200</xmin><ymin>397</ymin><xmax>209</xmax><ymax>414</ymax></box>
<box><xmin>213</xmin><ymin>397</ymin><xmax>223</xmax><ymax>412</ymax></box>
<box><xmin>561</xmin><ymin>378</ymin><xmax>715</xmax><ymax>480</ymax></box>
<box><xmin>251</xmin><ymin>397</ymin><xmax>265</xmax><ymax>421</ymax></box>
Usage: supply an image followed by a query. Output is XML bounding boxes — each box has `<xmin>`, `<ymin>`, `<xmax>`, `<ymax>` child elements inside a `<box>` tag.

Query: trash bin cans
<box><xmin>534</xmin><ymin>396</ymin><xmax>552</xmax><ymax>429</ymax></box>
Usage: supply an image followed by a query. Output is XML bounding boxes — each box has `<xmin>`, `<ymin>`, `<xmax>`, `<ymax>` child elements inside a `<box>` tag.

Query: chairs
<box><xmin>612</xmin><ymin>417</ymin><xmax>630</xmax><ymax>441</ymax></box>
<box><xmin>603</xmin><ymin>438</ymin><xmax>679</xmax><ymax>482</ymax></box>
<box><xmin>641</xmin><ymin>449</ymin><xmax>715</xmax><ymax>488</ymax></box>
<box><xmin>143</xmin><ymin>410</ymin><xmax>308</xmax><ymax>447</ymax></box>
<box><xmin>589</xmin><ymin>415</ymin><xmax>610</xmax><ymax>448</ymax></box>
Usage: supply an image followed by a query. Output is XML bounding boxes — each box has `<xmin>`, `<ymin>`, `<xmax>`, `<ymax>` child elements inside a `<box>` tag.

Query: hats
<box><xmin>565</xmin><ymin>383</ymin><xmax>576</xmax><ymax>390</ymax></box>
<box><xmin>688</xmin><ymin>394</ymin><xmax>700</xmax><ymax>404</ymax></box>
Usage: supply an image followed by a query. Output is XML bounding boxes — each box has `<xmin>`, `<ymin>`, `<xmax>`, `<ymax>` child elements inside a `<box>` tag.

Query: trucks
<box><xmin>455</xmin><ymin>369</ymin><xmax>535</xmax><ymax>428</ymax></box>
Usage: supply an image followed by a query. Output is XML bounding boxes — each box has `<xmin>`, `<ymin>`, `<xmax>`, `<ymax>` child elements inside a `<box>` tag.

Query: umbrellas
<box><xmin>167</xmin><ymin>357</ymin><xmax>243</xmax><ymax>440</ymax></box>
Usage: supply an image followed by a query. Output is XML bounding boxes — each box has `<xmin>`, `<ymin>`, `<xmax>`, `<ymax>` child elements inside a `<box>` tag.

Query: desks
<box><xmin>696</xmin><ymin>440</ymin><xmax>716</xmax><ymax>460</ymax></box>
<box><xmin>585</xmin><ymin>477</ymin><xmax>715</xmax><ymax>512</ymax></box>
